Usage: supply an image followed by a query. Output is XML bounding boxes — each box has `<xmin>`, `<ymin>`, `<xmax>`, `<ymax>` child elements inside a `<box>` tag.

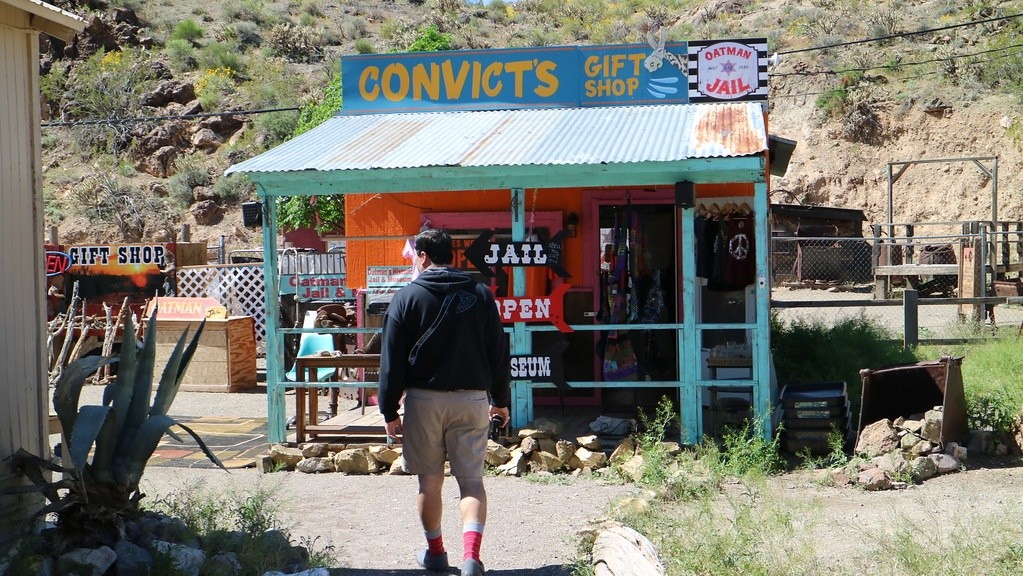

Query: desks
<box><xmin>292</xmin><ymin>353</ymin><xmax>406</xmax><ymax>442</ymax></box>
<box><xmin>706</xmin><ymin>355</ymin><xmax>754</xmax><ymax>435</ymax></box>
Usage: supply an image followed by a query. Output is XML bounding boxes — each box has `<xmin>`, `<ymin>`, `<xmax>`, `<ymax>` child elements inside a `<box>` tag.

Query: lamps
<box><xmin>674</xmin><ymin>178</ymin><xmax>698</xmax><ymax>211</ymax></box>
<box><xmin>241</xmin><ymin>200</ymin><xmax>263</xmax><ymax>231</ymax></box>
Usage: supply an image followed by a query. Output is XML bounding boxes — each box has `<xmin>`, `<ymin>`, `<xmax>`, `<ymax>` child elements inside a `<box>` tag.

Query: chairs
<box><xmin>284</xmin><ymin>332</ymin><xmax>337</xmax><ymax>429</ymax></box>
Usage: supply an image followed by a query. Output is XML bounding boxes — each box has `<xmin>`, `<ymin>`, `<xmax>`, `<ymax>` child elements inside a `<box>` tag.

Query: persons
<box><xmin>378</xmin><ymin>228</ymin><xmax>510</xmax><ymax>576</ymax></box>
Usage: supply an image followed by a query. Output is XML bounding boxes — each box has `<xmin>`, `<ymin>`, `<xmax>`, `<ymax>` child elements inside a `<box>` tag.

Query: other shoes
<box><xmin>461</xmin><ymin>557</ymin><xmax>485</xmax><ymax>576</ymax></box>
<box><xmin>417</xmin><ymin>549</ymin><xmax>448</xmax><ymax>572</ymax></box>
